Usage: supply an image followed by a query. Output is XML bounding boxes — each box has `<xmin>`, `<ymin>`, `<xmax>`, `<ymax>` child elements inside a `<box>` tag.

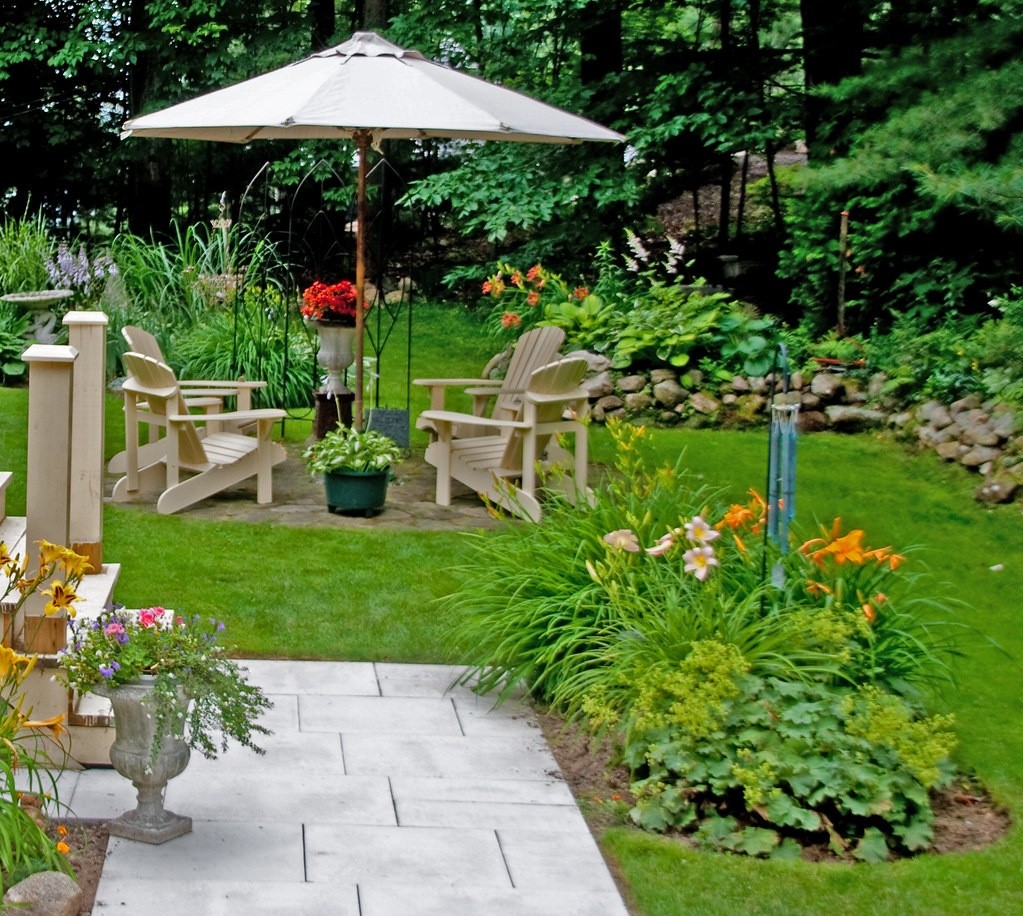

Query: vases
<box><xmin>103</xmin><ymin>672</ymin><xmax>192</xmax><ymax>843</ymax></box>
<box><xmin>317</xmin><ymin>319</ymin><xmax>356</xmax><ymax>394</ymax></box>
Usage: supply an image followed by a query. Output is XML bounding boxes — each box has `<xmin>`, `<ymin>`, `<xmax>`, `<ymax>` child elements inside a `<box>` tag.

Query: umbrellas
<box><xmin>119</xmin><ymin>29</ymin><xmax>629</xmax><ymax>434</ymax></box>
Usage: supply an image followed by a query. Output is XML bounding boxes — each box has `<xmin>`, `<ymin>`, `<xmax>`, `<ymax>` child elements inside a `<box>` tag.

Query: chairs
<box><xmin>411</xmin><ymin>324</ymin><xmax>574</xmax><ymax>474</ymax></box>
<box><xmin>111</xmin><ymin>352</ymin><xmax>290</xmax><ymax>516</ymax></box>
<box><xmin>419</xmin><ymin>356</ymin><xmax>600</xmax><ymax>522</ymax></box>
<box><xmin>107</xmin><ymin>326</ymin><xmax>267</xmax><ymax>473</ymax></box>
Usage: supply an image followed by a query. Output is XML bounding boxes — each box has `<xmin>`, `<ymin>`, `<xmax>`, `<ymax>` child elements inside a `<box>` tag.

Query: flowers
<box><xmin>299</xmin><ymin>281</ymin><xmax>372</xmax><ymax>324</ymax></box>
<box><xmin>51</xmin><ymin>608</ymin><xmax>274</xmax><ymax>775</ymax></box>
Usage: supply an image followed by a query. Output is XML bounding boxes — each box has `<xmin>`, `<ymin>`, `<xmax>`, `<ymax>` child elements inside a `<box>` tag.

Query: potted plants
<box><xmin>302</xmin><ymin>420</ymin><xmax>405</xmax><ymax>517</ymax></box>
